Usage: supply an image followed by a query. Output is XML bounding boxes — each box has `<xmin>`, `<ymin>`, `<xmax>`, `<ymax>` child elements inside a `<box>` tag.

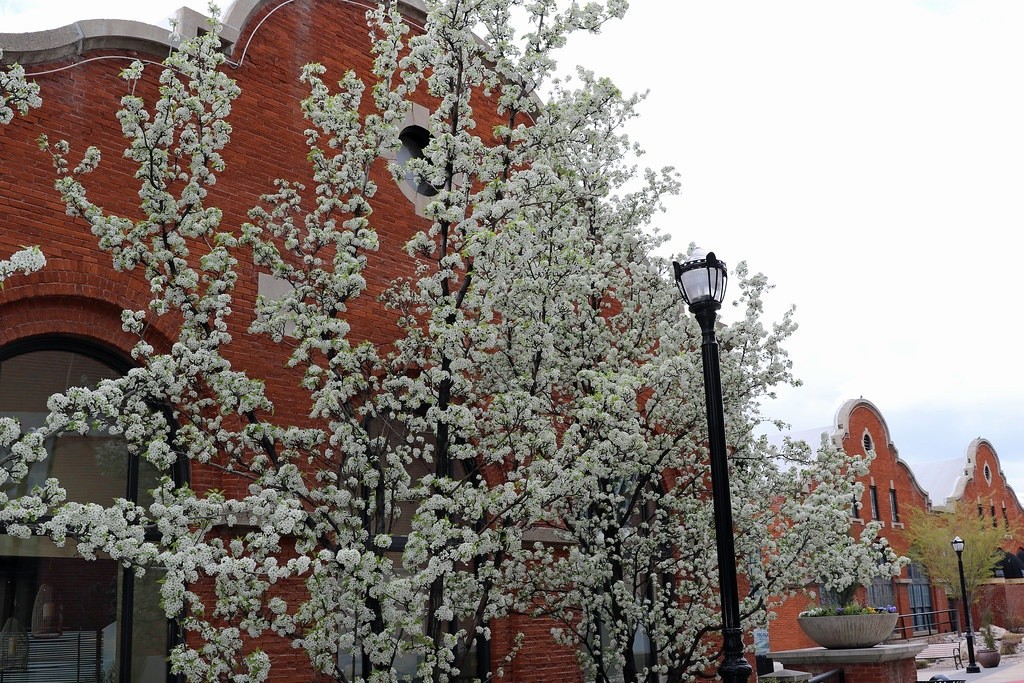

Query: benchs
<box><xmin>915</xmin><ymin>642</ymin><xmax>964</xmax><ymax>670</ymax></box>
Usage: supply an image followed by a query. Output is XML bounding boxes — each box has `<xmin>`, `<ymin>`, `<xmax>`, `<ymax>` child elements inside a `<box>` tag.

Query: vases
<box><xmin>797</xmin><ymin>613</ymin><xmax>900</xmax><ymax>649</ymax></box>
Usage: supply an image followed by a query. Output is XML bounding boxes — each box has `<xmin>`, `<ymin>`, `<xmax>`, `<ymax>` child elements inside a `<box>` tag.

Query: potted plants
<box><xmin>977</xmin><ymin>611</ymin><xmax>1000</xmax><ymax>668</ymax></box>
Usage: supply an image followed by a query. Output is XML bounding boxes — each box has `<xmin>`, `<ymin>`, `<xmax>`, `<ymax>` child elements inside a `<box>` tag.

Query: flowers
<box><xmin>799</xmin><ymin>601</ymin><xmax>897</xmax><ymax>618</ymax></box>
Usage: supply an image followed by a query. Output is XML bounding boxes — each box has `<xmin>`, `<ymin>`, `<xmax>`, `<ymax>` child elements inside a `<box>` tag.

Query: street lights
<box><xmin>672</xmin><ymin>247</ymin><xmax>753</xmax><ymax>683</ymax></box>
<box><xmin>950</xmin><ymin>535</ymin><xmax>981</xmax><ymax>673</ymax></box>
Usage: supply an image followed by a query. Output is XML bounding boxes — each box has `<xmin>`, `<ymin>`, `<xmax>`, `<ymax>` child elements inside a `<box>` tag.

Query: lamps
<box><xmin>0</xmin><ymin>618</ymin><xmax>29</xmax><ymax>672</ymax></box>
<box><xmin>31</xmin><ymin>584</ymin><xmax>62</xmax><ymax>639</ymax></box>
